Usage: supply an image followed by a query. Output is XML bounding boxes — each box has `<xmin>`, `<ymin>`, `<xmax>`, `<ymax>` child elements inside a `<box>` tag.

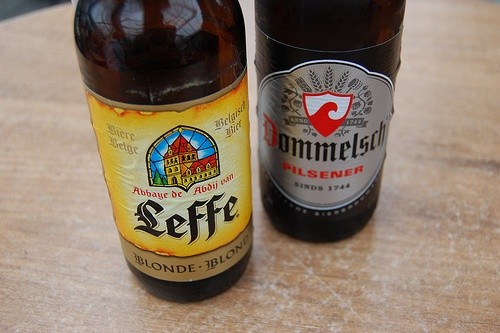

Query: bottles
<box><xmin>71</xmin><ymin>0</ymin><xmax>253</xmax><ymax>305</ymax></box>
<box><xmin>250</xmin><ymin>0</ymin><xmax>406</xmax><ymax>247</ymax></box>
